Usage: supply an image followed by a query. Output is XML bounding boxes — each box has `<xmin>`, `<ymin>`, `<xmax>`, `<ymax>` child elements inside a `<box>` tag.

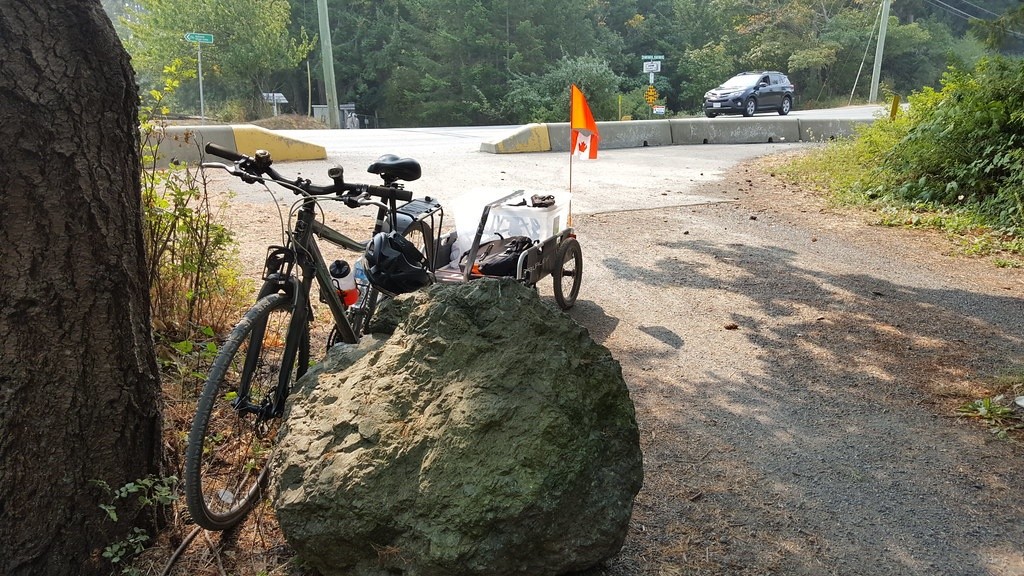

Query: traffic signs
<box><xmin>184</xmin><ymin>33</ymin><xmax>214</xmax><ymax>44</ymax></box>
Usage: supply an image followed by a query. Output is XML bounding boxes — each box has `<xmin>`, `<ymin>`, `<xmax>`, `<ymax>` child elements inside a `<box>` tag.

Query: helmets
<box><xmin>364</xmin><ymin>231</ymin><xmax>433</xmax><ymax>297</ymax></box>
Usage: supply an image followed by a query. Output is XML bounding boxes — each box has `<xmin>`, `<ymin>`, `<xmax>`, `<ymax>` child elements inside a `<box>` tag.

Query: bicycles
<box><xmin>185</xmin><ymin>142</ymin><xmax>450</xmax><ymax>532</ymax></box>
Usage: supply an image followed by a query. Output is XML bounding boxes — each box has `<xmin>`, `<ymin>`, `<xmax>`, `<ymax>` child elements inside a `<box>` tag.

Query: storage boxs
<box><xmin>446</xmin><ymin>186</ymin><xmax>574</xmax><ymax>256</ymax></box>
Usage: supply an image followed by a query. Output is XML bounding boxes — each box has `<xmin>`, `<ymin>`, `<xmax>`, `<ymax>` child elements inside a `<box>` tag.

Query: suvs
<box><xmin>702</xmin><ymin>69</ymin><xmax>795</xmax><ymax>118</ymax></box>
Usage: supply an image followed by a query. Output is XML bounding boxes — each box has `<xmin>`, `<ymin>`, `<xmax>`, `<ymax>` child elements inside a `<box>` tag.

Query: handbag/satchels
<box><xmin>531</xmin><ymin>194</ymin><xmax>555</xmax><ymax>206</ymax></box>
<box><xmin>459</xmin><ymin>232</ymin><xmax>540</xmax><ymax>276</ymax></box>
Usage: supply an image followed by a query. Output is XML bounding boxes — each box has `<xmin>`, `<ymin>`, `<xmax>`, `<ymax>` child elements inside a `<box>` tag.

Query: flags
<box><xmin>570</xmin><ymin>84</ymin><xmax>600</xmax><ymax>161</ymax></box>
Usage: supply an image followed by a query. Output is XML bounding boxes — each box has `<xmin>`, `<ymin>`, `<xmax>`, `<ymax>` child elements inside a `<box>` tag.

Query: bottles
<box><xmin>353</xmin><ymin>256</ymin><xmax>369</xmax><ymax>309</ymax></box>
<box><xmin>330</xmin><ymin>260</ymin><xmax>359</xmax><ymax>306</ymax></box>
